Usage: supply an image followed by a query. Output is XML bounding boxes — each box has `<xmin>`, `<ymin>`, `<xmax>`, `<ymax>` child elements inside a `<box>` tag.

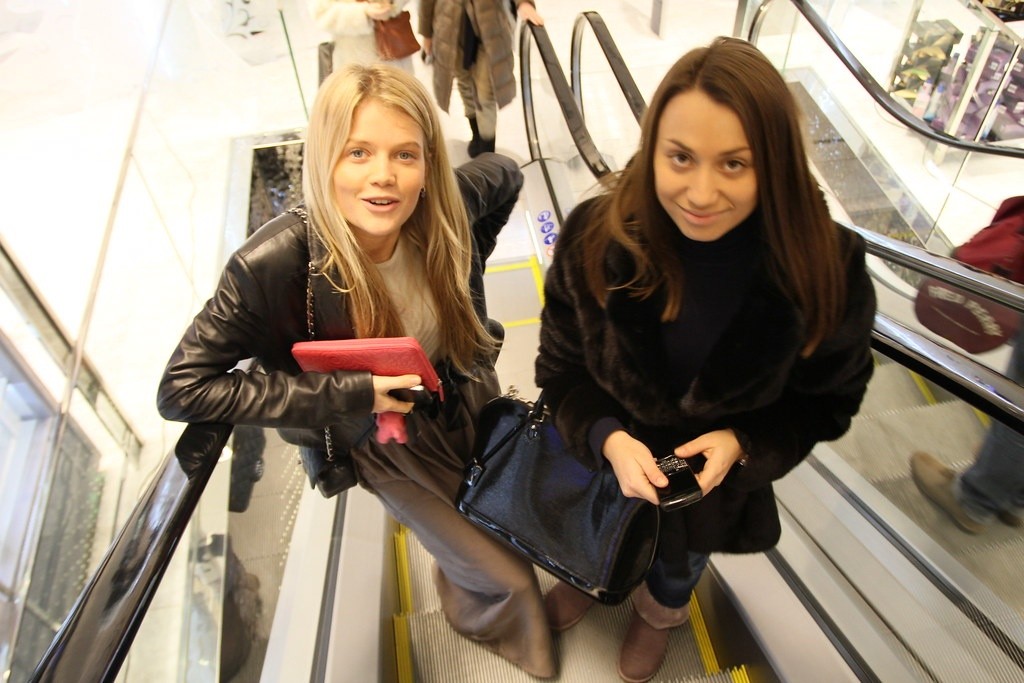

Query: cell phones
<box><xmin>654</xmin><ymin>454</ymin><xmax>703</xmax><ymax>512</ymax></box>
<box><xmin>387</xmin><ymin>385</ymin><xmax>435</xmax><ymax>405</ymax></box>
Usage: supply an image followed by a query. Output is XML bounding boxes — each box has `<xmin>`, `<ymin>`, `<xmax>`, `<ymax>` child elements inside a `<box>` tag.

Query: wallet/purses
<box><xmin>292</xmin><ymin>335</ymin><xmax>446</xmax><ymax>403</ymax></box>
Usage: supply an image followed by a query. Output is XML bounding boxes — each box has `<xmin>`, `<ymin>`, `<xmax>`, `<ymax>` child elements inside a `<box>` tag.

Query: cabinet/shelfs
<box><xmin>889</xmin><ymin>19</ymin><xmax>964</xmax><ymax>113</ymax></box>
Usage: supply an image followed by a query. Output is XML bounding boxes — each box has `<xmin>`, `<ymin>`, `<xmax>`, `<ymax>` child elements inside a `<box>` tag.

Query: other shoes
<box><xmin>1000</xmin><ymin>502</ymin><xmax>1024</xmax><ymax>528</ymax></box>
<box><xmin>910</xmin><ymin>450</ymin><xmax>994</xmax><ymax>536</ymax></box>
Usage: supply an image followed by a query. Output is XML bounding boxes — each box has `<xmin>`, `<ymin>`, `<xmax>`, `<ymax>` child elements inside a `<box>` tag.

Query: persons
<box><xmin>309</xmin><ymin>0</ymin><xmax>415</xmax><ymax>74</ymax></box>
<box><xmin>911</xmin><ymin>195</ymin><xmax>1024</xmax><ymax>532</ymax></box>
<box><xmin>418</xmin><ymin>0</ymin><xmax>544</xmax><ymax>158</ymax></box>
<box><xmin>156</xmin><ymin>62</ymin><xmax>555</xmax><ymax>675</ymax></box>
<box><xmin>534</xmin><ymin>35</ymin><xmax>876</xmax><ymax>682</ymax></box>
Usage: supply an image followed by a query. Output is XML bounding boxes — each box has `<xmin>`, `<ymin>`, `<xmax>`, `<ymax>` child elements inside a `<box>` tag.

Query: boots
<box><xmin>544</xmin><ymin>571</ymin><xmax>595</xmax><ymax>629</ymax></box>
<box><xmin>468</xmin><ymin>115</ymin><xmax>496</xmax><ymax>158</ymax></box>
<box><xmin>620</xmin><ymin>579</ymin><xmax>690</xmax><ymax>683</ymax></box>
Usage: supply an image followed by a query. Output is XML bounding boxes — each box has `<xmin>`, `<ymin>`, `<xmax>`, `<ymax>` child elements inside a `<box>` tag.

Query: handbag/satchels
<box><xmin>452</xmin><ymin>378</ymin><xmax>655</xmax><ymax>606</ymax></box>
<box><xmin>374</xmin><ymin>10</ymin><xmax>421</xmax><ymax>61</ymax></box>
<box><xmin>299</xmin><ymin>443</ymin><xmax>357</xmax><ymax>497</ymax></box>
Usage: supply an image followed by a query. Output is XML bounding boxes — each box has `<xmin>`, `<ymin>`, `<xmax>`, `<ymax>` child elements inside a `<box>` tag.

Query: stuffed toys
<box><xmin>375</xmin><ymin>411</ymin><xmax>409</xmax><ymax>444</ymax></box>
